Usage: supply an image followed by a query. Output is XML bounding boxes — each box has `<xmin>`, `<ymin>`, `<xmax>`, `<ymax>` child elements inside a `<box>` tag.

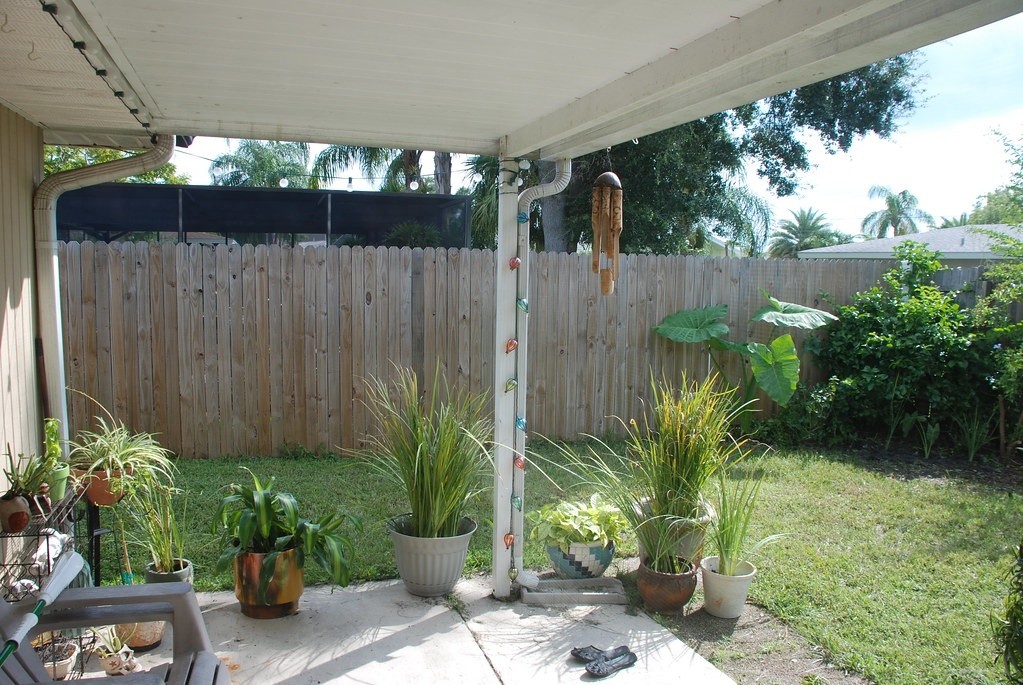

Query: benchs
<box><xmin>0</xmin><ymin>582</ymin><xmax>231</xmax><ymax>685</ymax></box>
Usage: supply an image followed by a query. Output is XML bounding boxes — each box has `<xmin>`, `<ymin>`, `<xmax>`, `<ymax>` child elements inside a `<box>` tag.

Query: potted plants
<box><xmin>685</xmin><ymin>450</ymin><xmax>792</xmax><ymax>618</ymax></box>
<box><xmin>126</xmin><ymin>476</ymin><xmax>194</xmax><ymax>584</ymax></box>
<box><xmin>72</xmin><ymin>409</ymin><xmax>183</xmax><ymax>505</ymax></box>
<box><xmin>522</xmin><ymin>492</ymin><xmax>627</xmax><ymax>580</ymax></box>
<box><xmin>584</xmin><ymin>371</ymin><xmax>745</xmax><ymax>615</ymax></box>
<box><xmin>33</xmin><ymin>416</ymin><xmax>70</xmax><ymax>501</ymax></box>
<box><xmin>210</xmin><ymin>466</ymin><xmax>364</xmax><ymax>620</ymax></box>
<box><xmin>337</xmin><ymin>357</ymin><xmax>505</xmax><ymax>597</ymax></box>
<box><xmin>4</xmin><ymin>441</ymin><xmax>55</xmax><ymax>511</ymax></box>
<box><xmin>630</xmin><ymin>442</ymin><xmax>712</xmax><ymax>568</ymax></box>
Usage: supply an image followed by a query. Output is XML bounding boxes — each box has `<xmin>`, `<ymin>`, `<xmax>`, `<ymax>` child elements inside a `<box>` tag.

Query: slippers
<box><xmin>571</xmin><ymin>645</ymin><xmax>630</xmax><ymax>663</ymax></box>
<box><xmin>585</xmin><ymin>652</ymin><xmax>638</xmax><ymax>677</ymax></box>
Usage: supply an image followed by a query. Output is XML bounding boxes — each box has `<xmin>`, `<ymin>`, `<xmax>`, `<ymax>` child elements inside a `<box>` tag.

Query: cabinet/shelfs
<box><xmin>0</xmin><ymin>482</ymin><xmax>101</xmax><ymax>602</ymax></box>
